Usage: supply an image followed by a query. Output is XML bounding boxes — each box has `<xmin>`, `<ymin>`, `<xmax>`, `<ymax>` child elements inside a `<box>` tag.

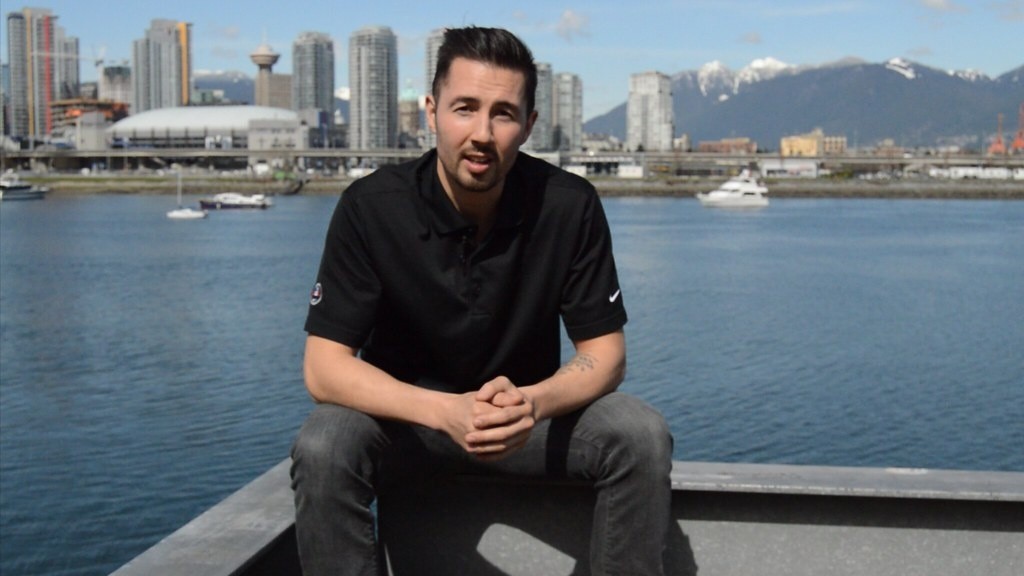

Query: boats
<box><xmin>200</xmin><ymin>191</ymin><xmax>274</xmax><ymax>209</ymax></box>
<box><xmin>0</xmin><ymin>174</ymin><xmax>52</xmax><ymax>200</ymax></box>
<box><xmin>165</xmin><ymin>165</ymin><xmax>208</xmax><ymax>220</ymax></box>
<box><xmin>696</xmin><ymin>175</ymin><xmax>770</xmax><ymax>208</ymax></box>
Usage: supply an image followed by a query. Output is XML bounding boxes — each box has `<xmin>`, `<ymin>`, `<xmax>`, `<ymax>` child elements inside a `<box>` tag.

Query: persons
<box><xmin>288</xmin><ymin>26</ymin><xmax>673</xmax><ymax>576</ymax></box>
